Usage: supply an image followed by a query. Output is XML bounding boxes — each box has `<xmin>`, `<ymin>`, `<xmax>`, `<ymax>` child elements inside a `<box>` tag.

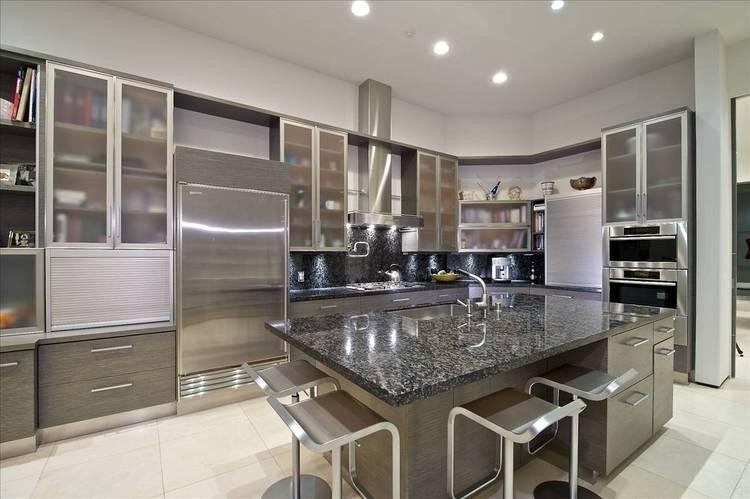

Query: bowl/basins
<box><xmin>570</xmin><ymin>176</ymin><xmax>598</xmax><ymax>189</ymax></box>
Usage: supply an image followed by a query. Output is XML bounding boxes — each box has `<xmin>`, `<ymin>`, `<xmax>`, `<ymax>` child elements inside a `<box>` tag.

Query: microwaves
<box><xmin>347</xmin><ymin>280</ymin><xmax>413</xmax><ymax>291</ymax></box>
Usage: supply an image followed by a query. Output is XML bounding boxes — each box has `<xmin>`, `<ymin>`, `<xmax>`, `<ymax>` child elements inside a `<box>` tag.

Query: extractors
<box><xmin>347</xmin><ymin>79</ymin><xmax>425</xmax><ymax>228</ymax></box>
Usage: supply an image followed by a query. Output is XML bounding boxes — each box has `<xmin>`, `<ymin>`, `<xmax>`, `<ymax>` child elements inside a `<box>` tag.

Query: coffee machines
<box><xmin>491</xmin><ymin>257</ymin><xmax>512</xmax><ymax>283</ymax></box>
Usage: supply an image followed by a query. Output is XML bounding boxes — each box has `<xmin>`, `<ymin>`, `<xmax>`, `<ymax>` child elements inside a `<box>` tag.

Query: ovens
<box><xmin>600</xmin><ymin>223</ymin><xmax>691</xmax><ymax>315</ymax></box>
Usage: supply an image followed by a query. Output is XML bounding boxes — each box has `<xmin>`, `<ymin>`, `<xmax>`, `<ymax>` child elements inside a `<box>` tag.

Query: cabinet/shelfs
<box><xmin>36</xmin><ymin>327</ymin><xmax>179</xmax><ymax>447</ymax></box>
<box><xmin>269</xmin><ymin>109</ymin><xmax>350</xmax><ymax>255</ymax></box>
<box><xmin>0</xmin><ymin>42</ymin><xmax>45</xmax><ymax>339</ymax></box>
<box><xmin>288</xmin><ymin>298</ymin><xmax>363</xmax><ymax>321</ymax></box>
<box><xmin>652</xmin><ymin>310</ymin><xmax>677</xmax><ymax>440</ymax></box>
<box><xmin>0</xmin><ymin>333</ymin><xmax>40</xmax><ymax>460</ymax></box>
<box><xmin>459</xmin><ymin>197</ymin><xmax>535</xmax><ymax>256</ymax></box>
<box><xmin>528</xmin><ymin>284</ymin><xmax>603</xmax><ymax>302</ymax></box>
<box><xmin>46</xmin><ymin>50</ymin><xmax>178</xmax><ymax>251</ymax></box>
<box><xmin>560</xmin><ymin>322</ymin><xmax>657</xmax><ymax>480</ymax></box>
<box><xmin>400</xmin><ymin>145</ymin><xmax>462</xmax><ymax>255</ymax></box>
<box><xmin>363</xmin><ymin>288</ymin><xmax>437</xmax><ymax>313</ymax></box>
<box><xmin>598</xmin><ymin>104</ymin><xmax>695</xmax><ymax>225</ymax></box>
<box><xmin>433</xmin><ymin>288</ymin><xmax>473</xmax><ymax>304</ymax></box>
<box><xmin>533</xmin><ymin>201</ymin><xmax>546</xmax><ymax>254</ymax></box>
<box><xmin>470</xmin><ymin>283</ymin><xmax>533</xmax><ymax>299</ymax></box>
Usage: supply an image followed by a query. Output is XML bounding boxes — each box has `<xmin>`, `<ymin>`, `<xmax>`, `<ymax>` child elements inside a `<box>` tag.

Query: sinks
<box><xmin>382</xmin><ymin>298</ymin><xmax>479</xmax><ymax>321</ymax></box>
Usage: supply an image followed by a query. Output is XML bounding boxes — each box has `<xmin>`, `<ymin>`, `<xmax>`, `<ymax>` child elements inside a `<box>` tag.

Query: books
<box><xmin>463</xmin><ymin>206</ymin><xmax>545</xmax><ymax>251</ymax></box>
<box><xmin>10</xmin><ymin>62</ymin><xmax>136</xmax><ymax>134</ymax></box>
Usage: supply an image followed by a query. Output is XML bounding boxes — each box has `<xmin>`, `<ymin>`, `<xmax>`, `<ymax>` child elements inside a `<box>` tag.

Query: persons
<box><xmin>18</xmin><ymin>235</ymin><xmax>29</xmax><ymax>248</ymax></box>
<box><xmin>15</xmin><ymin>165</ymin><xmax>30</xmax><ymax>185</ymax></box>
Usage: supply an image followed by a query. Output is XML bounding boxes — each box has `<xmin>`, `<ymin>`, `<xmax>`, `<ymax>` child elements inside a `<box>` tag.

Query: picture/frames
<box><xmin>14</xmin><ymin>161</ymin><xmax>36</xmax><ymax>188</ymax></box>
<box><xmin>6</xmin><ymin>228</ymin><xmax>37</xmax><ymax>249</ymax></box>
<box><xmin>0</xmin><ymin>163</ymin><xmax>18</xmax><ymax>186</ymax></box>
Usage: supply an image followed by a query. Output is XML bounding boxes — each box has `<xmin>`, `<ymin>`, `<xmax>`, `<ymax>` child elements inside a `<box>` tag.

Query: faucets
<box><xmin>453</xmin><ymin>314</ymin><xmax>487</xmax><ymax>348</ymax></box>
<box><xmin>455</xmin><ymin>267</ymin><xmax>490</xmax><ymax>315</ymax></box>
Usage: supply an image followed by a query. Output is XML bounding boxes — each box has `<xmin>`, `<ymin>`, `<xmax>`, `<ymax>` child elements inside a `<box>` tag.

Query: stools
<box><xmin>522</xmin><ymin>356</ymin><xmax>636</xmax><ymax>499</ymax></box>
<box><xmin>239</xmin><ymin>356</ymin><xmax>343</xmax><ymax>499</ymax></box>
<box><xmin>265</xmin><ymin>391</ymin><xmax>402</xmax><ymax>499</ymax></box>
<box><xmin>442</xmin><ymin>385</ymin><xmax>581</xmax><ymax>498</ymax></box>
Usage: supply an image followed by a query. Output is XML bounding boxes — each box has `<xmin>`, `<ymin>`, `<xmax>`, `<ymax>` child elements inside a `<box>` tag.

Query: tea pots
<box><xmin>385</xmin><ymin>263</ymin><xmax>404</xmax><ymax>283</ymax></box>
